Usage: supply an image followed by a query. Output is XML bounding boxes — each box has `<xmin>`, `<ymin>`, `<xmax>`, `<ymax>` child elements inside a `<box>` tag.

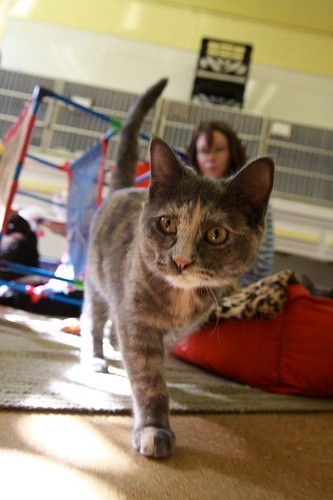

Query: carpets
<box><xmin>0</xmin><ymin>313</ymin><xmax>333</xmax><ymax>414</ymax></box>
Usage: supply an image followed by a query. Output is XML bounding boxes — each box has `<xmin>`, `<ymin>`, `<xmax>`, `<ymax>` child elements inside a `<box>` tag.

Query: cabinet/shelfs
<box><xmin>1</xmin><ymin>67</ymin><xmax>333</xmax><ymax>264</ymax></box>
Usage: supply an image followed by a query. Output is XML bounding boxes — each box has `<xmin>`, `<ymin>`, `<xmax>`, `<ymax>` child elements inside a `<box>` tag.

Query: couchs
<box><xmin>171</xmin><ymin>287</ymin><xmax>333</xmax><ymax>402</ymax></box>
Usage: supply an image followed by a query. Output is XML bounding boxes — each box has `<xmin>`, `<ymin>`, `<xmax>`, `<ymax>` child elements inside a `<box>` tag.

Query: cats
<box><xmin>79</xmin><ymin>78</ymin><xmax>275</xmax><ymax>458</ymax></box>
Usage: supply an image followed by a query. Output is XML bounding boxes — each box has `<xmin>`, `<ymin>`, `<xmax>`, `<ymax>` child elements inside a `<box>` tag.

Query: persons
<box><xmin>1</xmin><ymin>210</ymin><xmax>42</xmax><ymax>269</ymax></box>
<box><xmin>188</xmin><ymin>119</ymin><xmax>275</xmax><ymax>301</ymax></box>
